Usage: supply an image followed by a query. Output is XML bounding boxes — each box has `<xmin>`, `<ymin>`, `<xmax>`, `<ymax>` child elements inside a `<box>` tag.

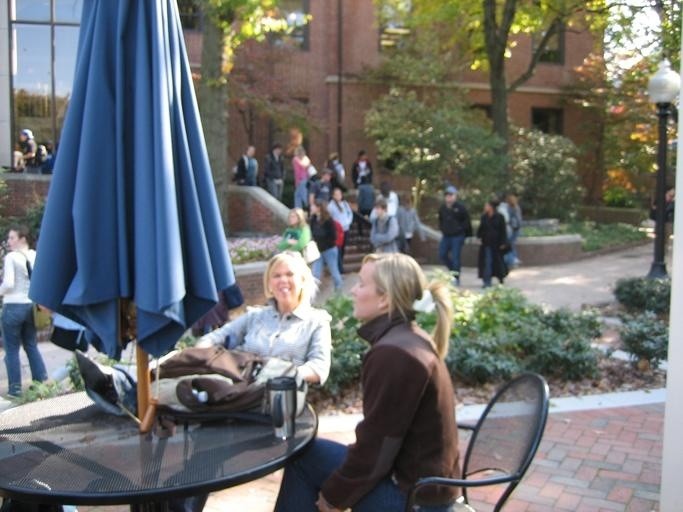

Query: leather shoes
<box><xmin>72</xmin><ymin>348</ymin><xmax>122</xmax><ymax>410</ymax></box>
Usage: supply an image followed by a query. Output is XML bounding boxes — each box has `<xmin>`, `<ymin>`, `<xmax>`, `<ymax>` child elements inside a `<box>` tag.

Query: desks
<box><xmin>0</xmin><ymin>388</ymin><xmax>318</xmax><ymax>512</ymax></box>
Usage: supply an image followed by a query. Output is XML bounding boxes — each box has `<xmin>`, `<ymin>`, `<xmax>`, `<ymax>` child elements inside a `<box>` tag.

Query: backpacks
<box><xmin>142</xmin><ymin>347</ymin><xmax>310</xmax><ymax>427</ymax></box>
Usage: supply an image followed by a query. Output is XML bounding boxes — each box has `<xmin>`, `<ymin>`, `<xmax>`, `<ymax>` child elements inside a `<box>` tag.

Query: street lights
<box><xmin>645</xmin><ymin>55</ymin><xmax>681</xmax><ymax>278</ymax></box>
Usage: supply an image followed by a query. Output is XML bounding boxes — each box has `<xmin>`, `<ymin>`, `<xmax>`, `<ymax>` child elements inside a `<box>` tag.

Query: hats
<box><xmin>443</xmin><ymin>186</ymin><xmax>456</xmax><ymax>194</ymax></box>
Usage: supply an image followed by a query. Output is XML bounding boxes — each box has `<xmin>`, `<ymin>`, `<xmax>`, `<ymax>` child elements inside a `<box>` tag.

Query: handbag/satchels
<box><xmin>301</xmin><ymin>240</ymin><xmax>320</xmax><ymax>264</ymax></box>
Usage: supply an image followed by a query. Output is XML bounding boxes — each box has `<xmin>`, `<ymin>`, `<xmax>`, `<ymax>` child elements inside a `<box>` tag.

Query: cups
<box><xmin>266</xmin><ymin>376</ymin><xmax>299</xmax><ymax>443</ymax></box>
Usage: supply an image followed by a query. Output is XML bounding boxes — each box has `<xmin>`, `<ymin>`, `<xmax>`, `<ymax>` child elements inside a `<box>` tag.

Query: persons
<box><xmin>438</xmin><ymin>179</ymin><xmax>525</xmax><ymax>293</ymax></box>
<box><xmin>0</xmin><ymin>222</ymin><xmax>49</xmax><ymax>399</ymax></box>
<box><xmin>12</xmin><ymin>126</ymin><xmax>37</xmax><ymax>173</ymax></box>
<box><xmin>271</xmin><ymin>251</ymin><xmax>464</xmax><ymax>512</ymax></box>
<box><xmin>229</xmin><ymin>126</ymin><xmax>426</xmax><ymax>293</ymax></box>
<box><xmin>194</xmin><ymin>249</ymin><xmax>332</xmax><ymax>389</ymax></box>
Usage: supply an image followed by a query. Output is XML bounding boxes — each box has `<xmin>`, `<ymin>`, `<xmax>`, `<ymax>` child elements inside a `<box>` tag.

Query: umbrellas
<box><xmin>29</xmin><ymin>0</ymin><xmax>239</xmax><ymax>491</ymax></box>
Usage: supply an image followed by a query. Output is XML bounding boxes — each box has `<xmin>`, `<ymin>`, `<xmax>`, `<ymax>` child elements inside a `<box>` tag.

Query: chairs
<box><xmin>405</xmin><ymin>371</ymin><xmax>551</xmax><ymax>511</ymax></box>
